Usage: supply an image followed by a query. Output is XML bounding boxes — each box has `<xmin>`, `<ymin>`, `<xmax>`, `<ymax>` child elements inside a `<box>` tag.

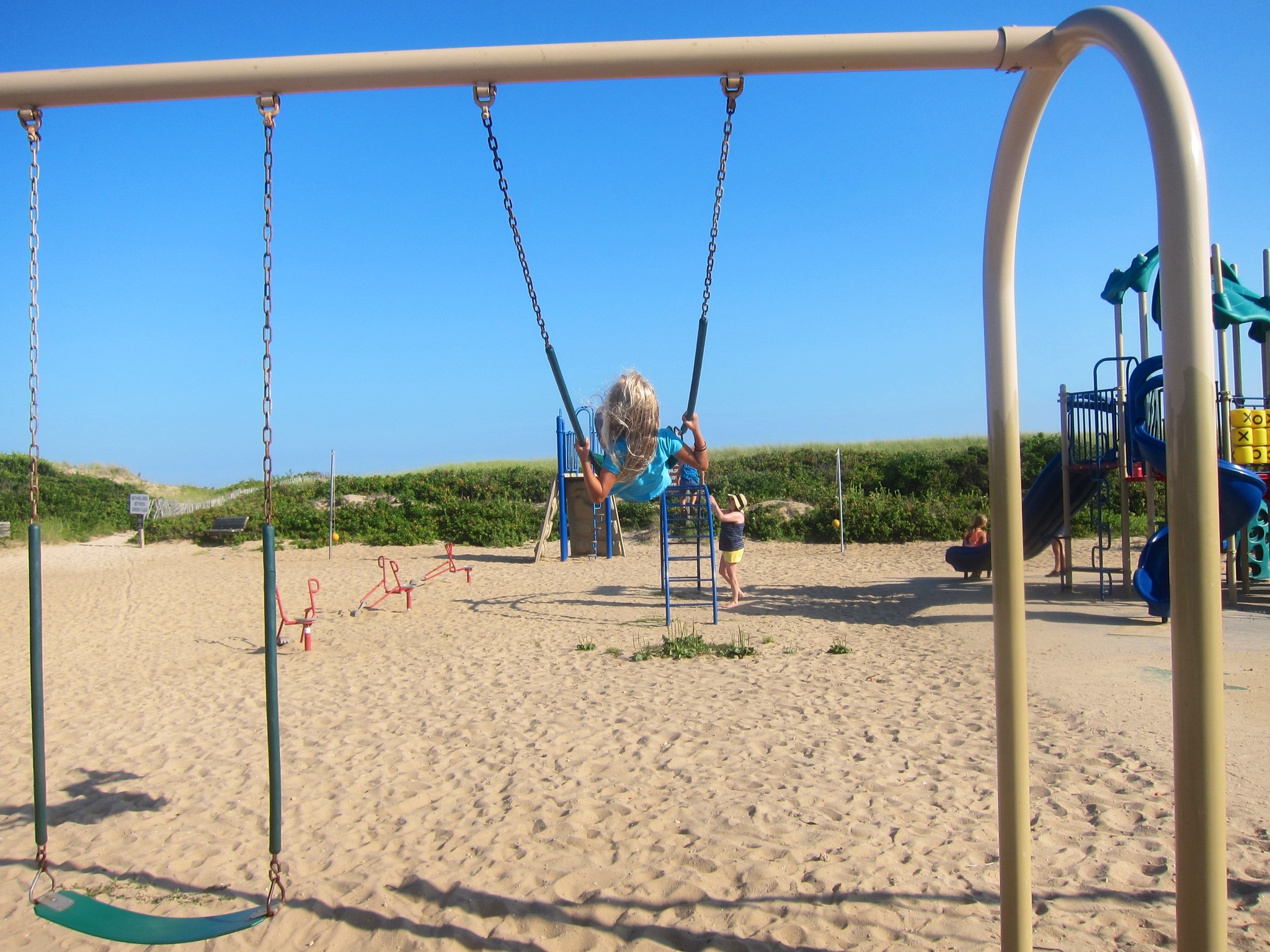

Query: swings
<box><xmin>17</xmin><ymin>93</ymin><xmax>286</xmax><ymax>945</ymax></box>
<box><xmin>470</xmin><ymin>73</ymin><xmax>749</xmax><ymax>502</ymax></box>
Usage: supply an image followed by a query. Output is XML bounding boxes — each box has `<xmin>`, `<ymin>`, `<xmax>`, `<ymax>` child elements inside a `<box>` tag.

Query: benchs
<box><xmin>204</xmin><ymin>516</ymin><xmax>249</xmax><ymax>541</ymax></box>
<box><xmin>0</xmin><ymin>521</ymin><xmax>11</xmax><ymax>547</ymax></box>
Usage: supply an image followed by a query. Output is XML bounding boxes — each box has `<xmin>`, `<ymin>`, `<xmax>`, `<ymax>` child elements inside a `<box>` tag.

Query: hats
<box><xmin>727</xmin><ymin>493</ymin><xmax>748</xmax><ymax>514</ymax></box>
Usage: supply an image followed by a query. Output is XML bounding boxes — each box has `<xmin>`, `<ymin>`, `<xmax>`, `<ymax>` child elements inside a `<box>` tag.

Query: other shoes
<box><xmin>1059</xmin><ymin>570</ymin><xmax>1067</xmax><ymax>575</ymax></box>
<box><xmin>1045</xmin><ymin>570</ymin><xmax>1060</xmax><ymax>577</ymax></box>
<box><xmin>682</xmin><ymin>509</ymin><xmax>688</xmax><ymax>518</ymax></box>
<box><xmin>690</xmin><ymin>506</ymin><xmax>695</xmax><ymax>516</ymax></box>
<box><xmin>594</xmin><ymin>405</ymin><xmax>605</xmax><ymax>441</ymax></box>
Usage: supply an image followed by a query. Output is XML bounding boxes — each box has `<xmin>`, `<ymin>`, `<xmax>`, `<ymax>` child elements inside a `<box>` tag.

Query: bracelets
<box><xmin>713</xmin><ymin>505</ymin><xmax>718</xmax><ymax>509</ymax></box>
<box><xmin>695</xmin><ymin>447</ymin><xmax>707</xmax><ymax>451</ymax></box>
<box><xmin>694</xmin><ymin>441</ymin><xmax>706</xmax><ymax>448</ymax></box>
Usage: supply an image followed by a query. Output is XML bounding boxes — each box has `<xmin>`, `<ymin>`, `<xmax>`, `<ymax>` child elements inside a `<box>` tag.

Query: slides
<box><xmin>945</xmin><ymin>448</ymin><xmax>1115</xmax><ymax>572</ymax></box>
<box><xmin>1127</xmin><ymin>352</ymin><xmax>1266</xmax><ymax>621</ymax></box>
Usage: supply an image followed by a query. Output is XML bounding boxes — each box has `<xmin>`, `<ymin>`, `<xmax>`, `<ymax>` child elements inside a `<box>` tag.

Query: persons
<box><xmin>1022</xmin><ymin>489</ymin><xmax>1068</xmax><ymax>577</ymax></box>
<box><xmin>963</xmin><ymin>515</ymin><xmax>987</xmax><ymax>578</ymax></box>
<box><xmin>574</xmin><ymin>370</ymin><xmax>709</xmax><ymax>504</ymax></box>
<box><xmin>702</xmin><ymin>491</ymin><xmax>748</xmax><ymax>608</ymax></box>
<box><xmin>676</xmin><ymin>446</ymin><xmax>704</xmax><ymax>518</ymax></box>
